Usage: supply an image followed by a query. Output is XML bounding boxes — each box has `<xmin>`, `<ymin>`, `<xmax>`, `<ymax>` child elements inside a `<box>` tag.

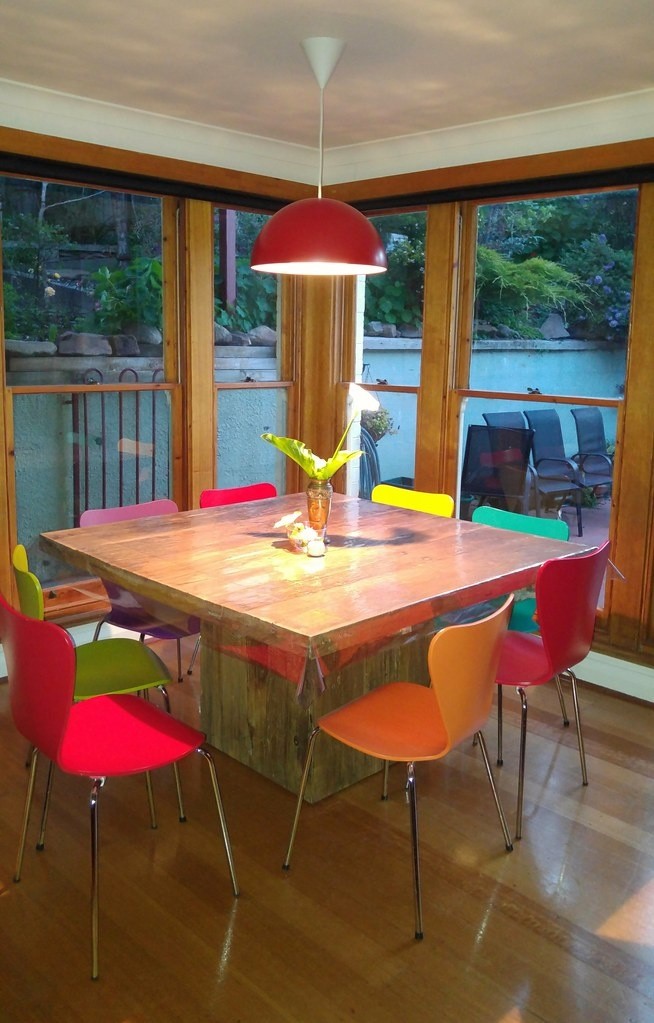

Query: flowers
<box><xmin>361</xmin><ymin>407</ymin><xmax>401</xmax><ymax>440</ymax></box>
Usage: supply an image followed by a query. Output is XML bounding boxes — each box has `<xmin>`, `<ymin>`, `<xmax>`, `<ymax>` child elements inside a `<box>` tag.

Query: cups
<box><xmin>285</xmin><ymin>521</ymin><xmax>326</xmax><ymax>554</ymax></box>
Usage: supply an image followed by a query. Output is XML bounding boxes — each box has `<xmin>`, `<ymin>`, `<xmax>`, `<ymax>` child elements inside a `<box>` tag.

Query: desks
<box><xmin>38</xmin><ymin>490</ymin><xmax>628</xmax><ymax>805</ymax></box>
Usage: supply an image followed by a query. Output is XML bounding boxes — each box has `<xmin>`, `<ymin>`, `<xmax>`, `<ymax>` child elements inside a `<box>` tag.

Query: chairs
<box><xmin>0</xmin><ymin>483</ymin><xmax>612</xmax><ymax>983</ymax></box>
<box><xmin>459</xmin><ymin>405</ymin><xmax>615</xmax><ymax>538</ymax></box>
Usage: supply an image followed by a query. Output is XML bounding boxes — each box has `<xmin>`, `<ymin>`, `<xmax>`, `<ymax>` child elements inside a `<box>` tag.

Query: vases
<box><xmin>364</xmin><ymin>426</ymin><xmax>387</xmax><ymax>443</ymax></box>
<box><xmin>306</xmin><ymin>479</ymin><xmax>334</xmax><ymax>543</ymax></box>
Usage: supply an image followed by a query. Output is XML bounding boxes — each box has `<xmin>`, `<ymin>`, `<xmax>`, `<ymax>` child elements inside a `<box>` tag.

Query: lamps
<box><xmin>249</xmin><ymin>37</ymin><xmax>388</xmax><ymax>275</ymax></box>
